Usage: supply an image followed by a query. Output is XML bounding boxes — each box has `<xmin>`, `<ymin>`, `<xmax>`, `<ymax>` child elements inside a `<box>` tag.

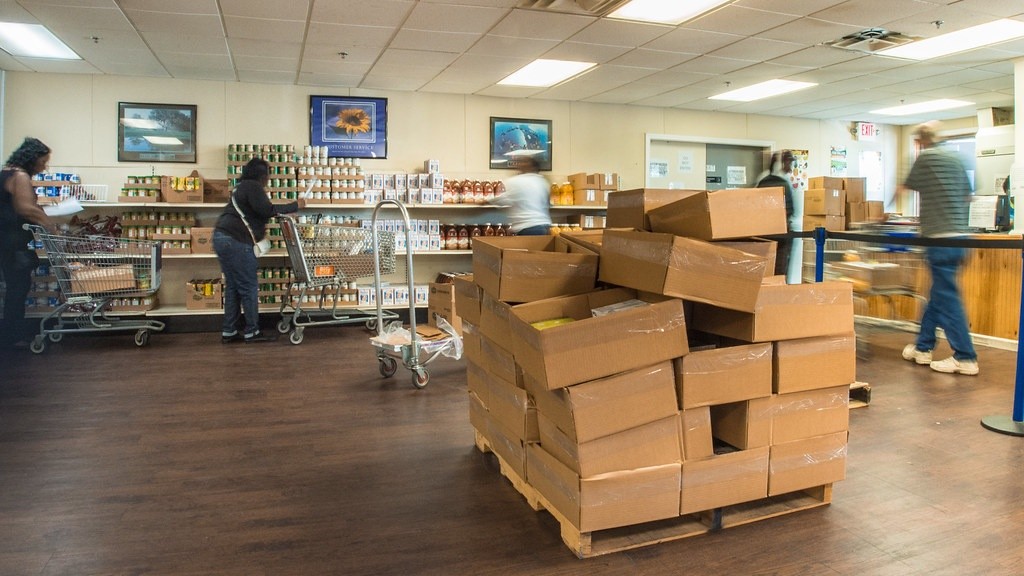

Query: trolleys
<box><xmin>272</xmin><ymin>214</ymin><xmax>402</xmax><ymax>344</ymax></box>
<box><xmin>20</xmin><ymin>222</ymin><xmax>167</xmax><ymax>355</ymax></box>
<box><xmin>367</xmin><ymin>199</ymin><xmax>466</xmax><ymax>387</ymax></box>
<box><xmin>802</xmin><ymin>227</ymin><xmax>939</xmax><ymax>359</ymax></box>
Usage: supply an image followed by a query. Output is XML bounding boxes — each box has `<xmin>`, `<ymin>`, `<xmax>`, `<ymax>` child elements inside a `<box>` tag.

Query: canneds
<box><xmin>121</xmin><ymin>175</ymin><xmax>195</xmax><ymax>250</ymax></box>
<box><xmin>257</xmin><ymin>215</ymin><xmax>359</xmax><ymax>302</ymax></box>
<box><xmin>110</xmin><ymin>294</ymin><xmax>157</xmax><ymax>306</ymax></box>
<box><xmin>186</xmin><ymin>279</ymin><xmax>221</xmax><ymax>298</ymax></box>
<box><xmin>24</xmin><ymin>172</ymin><xmax>80</xmax><ymax>306</ymax></box>
<box><xmin>228</xmin><ymin>144</ymin><xmax>365</xmax><ymax>199</ymax></box>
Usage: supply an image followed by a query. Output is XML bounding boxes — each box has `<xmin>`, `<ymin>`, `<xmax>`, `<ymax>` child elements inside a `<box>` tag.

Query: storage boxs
<box><xmin>33</xmin><ymin>169</ymin><xmax>885</xmax><ymax>533</ymax></box>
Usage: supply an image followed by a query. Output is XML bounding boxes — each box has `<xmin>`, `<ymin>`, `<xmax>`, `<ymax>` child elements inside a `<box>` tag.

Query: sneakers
<box><xmin>902</xmin><ymin>343</ymin><xmax>932</xmax><ymax>364</ymax></box>
<box><xmin>930</xmin><ymin>356</ymin><xmax>979</xmax><ymax>375</ymax></box>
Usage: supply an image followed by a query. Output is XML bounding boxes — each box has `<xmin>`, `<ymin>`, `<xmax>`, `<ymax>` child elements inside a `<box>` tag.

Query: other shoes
<box><xmin>0</xmin><ymin>340</ymin><xmax>30</xmax><ymax>350</ymax></box>
<box><xmin>245</xmin><ymin>330</ymin><xmax>275</xmax><ymax>341</ymax></box>
<box><xmin>222</xmin><ymin>332</ymin><xmax>244</xmax><ymax>344</ymax></box>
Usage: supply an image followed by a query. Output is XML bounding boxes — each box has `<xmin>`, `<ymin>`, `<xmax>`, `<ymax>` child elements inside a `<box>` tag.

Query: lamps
<box><xmin>851</xmin><ymin>122</ymin><xmax>880</xmax><ymax>142</ymax></box>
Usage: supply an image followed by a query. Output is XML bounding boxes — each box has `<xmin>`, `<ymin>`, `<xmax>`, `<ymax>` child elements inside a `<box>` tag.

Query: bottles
<box><xmin>440</xmin><ymin>178</ymin><xmax>582</xmax><ymax>249</ymax></box>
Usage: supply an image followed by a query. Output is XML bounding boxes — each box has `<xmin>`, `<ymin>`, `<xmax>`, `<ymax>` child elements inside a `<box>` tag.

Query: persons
<box><xmin>980</xmin><ymin>232</ymin><xmax>1023</xmax><ymax>438</ymax></box>
<box><xmin>487</xmin><ymin>158</ymin><xmax>550</xmax><ymax>235</ymax></box>
<box><xmin>995</xmin><ymin>175</ymin><xmax>1010</xmax><ymax>230</ymax></box>
<box><xmin>213</xmin><ymin>157</ymin><xmax>307</xmax><ymax>344</ymax></box>
<box><xmin>0</xmin><ymin>137</ymin><xmax>58</xmax><ymax>349</ymax></box>
<box><xmin>888</xmin><ymin>122</ymin><xmax>978</xmax><ymax>375</ymax></box>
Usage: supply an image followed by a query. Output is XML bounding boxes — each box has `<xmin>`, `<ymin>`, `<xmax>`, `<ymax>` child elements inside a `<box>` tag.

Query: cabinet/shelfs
<box><xmin>21</xmin><ymin>199</ymin><xmax>608</xmax><ymax>318</ymax></box>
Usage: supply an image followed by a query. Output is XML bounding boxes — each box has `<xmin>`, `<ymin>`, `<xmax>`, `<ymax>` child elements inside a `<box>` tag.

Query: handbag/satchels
<box><xmin>253</xmin><ymin>239</ymin><xmax>272</xmax><ymax>258</ymax></box>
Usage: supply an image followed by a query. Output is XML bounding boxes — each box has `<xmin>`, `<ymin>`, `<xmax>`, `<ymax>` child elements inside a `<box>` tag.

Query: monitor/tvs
<box><xmin>994</xmin><ymin>194</ymin><xmax>1011</xmax><ymax>227</ymax></box>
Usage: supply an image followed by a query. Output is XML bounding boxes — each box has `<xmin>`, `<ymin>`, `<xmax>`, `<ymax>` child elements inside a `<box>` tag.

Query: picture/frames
<box><xmin>490</xmin><ymin>117</ymin><xmax>553</xmax><ymax>172</ymax></box>
<box><xmin>118</xmin><ymin>102</ymin><xmax>197</xmax><ymax>163</ymax></box>
<box><xmin>310</xmin><ymin>94</ymin><xmax>387</xmax><ymax>159</ymax></box>
